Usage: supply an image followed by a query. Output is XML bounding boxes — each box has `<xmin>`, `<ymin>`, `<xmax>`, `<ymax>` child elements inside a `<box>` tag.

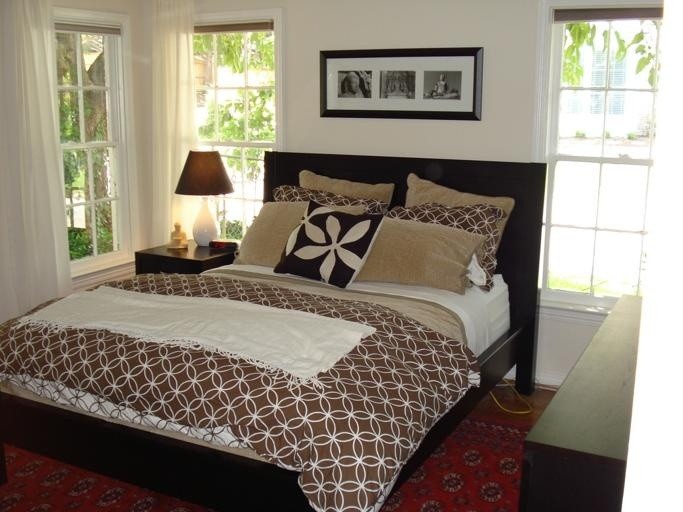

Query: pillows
<box><xmin>233</xmin><ymin>202</ymin><xmax>363</xmax><ymax>268</ymax></box>
<box><xmin>354</xmin><ymin>216</ymin><xmax>486</xmax><ymax>295</ymax></box>
<box><xmin>299</xmin><ymin>170</ymin><xmax>396</xmax><ymax>204</ymax></box>
<box><xmin>404</xmin><ymin>172</ymin><xmax>516</xmax><ymax>256</ymax></box>
<box><xmin>272</xmin><ymin>185</ymin><xmax>390</xmax><ymax>216</ymax></box>
<box><xmin>272</xmin><ymin>199</ymin><xmax>384</xmax><ymax>291</ymax></box>
<box><xmin>384</xmin><ymin>201</ymin><xmax>507</xmax><ymax>293</ymax></box>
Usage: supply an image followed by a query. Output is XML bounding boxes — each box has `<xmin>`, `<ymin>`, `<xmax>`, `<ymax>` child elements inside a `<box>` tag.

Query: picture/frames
<box><xmin>319</xmin><ymin>47</ymin><xmax>483</xmax><ymax>121</ymax></box>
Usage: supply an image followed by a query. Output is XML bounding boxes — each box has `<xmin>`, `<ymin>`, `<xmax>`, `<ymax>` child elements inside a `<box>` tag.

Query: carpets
<box><xmin>0</xmin><ymin>417</ymin><xmax>529</xmax><ymax>511</ymax></box>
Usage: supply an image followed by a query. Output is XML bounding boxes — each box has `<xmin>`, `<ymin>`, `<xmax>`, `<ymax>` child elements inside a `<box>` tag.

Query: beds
<box><xmin>0</xmin><ymin>151</ymin><xmax>548</xmax><ymax>511</ymax></box>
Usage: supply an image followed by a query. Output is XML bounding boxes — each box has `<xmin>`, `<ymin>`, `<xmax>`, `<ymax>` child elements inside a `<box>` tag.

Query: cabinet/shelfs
<box><xmin>518</xmin><ymin>294</ymin><xmax>642</xmax><ymax>511</ymax></box>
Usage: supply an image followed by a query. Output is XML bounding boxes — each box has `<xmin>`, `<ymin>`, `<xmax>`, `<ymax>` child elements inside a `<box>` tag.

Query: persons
<box><xmin>431</xmin><ymin>72</ymin><xmax>448</xmax><ymax>98</ymax></box>
<box><xmin>339</xmin><ymin>70</ymin><xmax>364</xmax><ymax>98</ymax></box>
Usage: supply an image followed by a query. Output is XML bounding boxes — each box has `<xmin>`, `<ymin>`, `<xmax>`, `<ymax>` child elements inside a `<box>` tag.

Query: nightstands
<box><xmin>134</xmin><ymin>239</ymin><xmax>238</xmax><ymax>275</ymax></box>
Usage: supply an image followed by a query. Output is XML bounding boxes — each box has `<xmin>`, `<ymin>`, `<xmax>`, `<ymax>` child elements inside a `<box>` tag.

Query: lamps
<box><xmin>174</xmin><ymin>150</ymin><xmax>236</xmax><ymax>247</ymax></box>
